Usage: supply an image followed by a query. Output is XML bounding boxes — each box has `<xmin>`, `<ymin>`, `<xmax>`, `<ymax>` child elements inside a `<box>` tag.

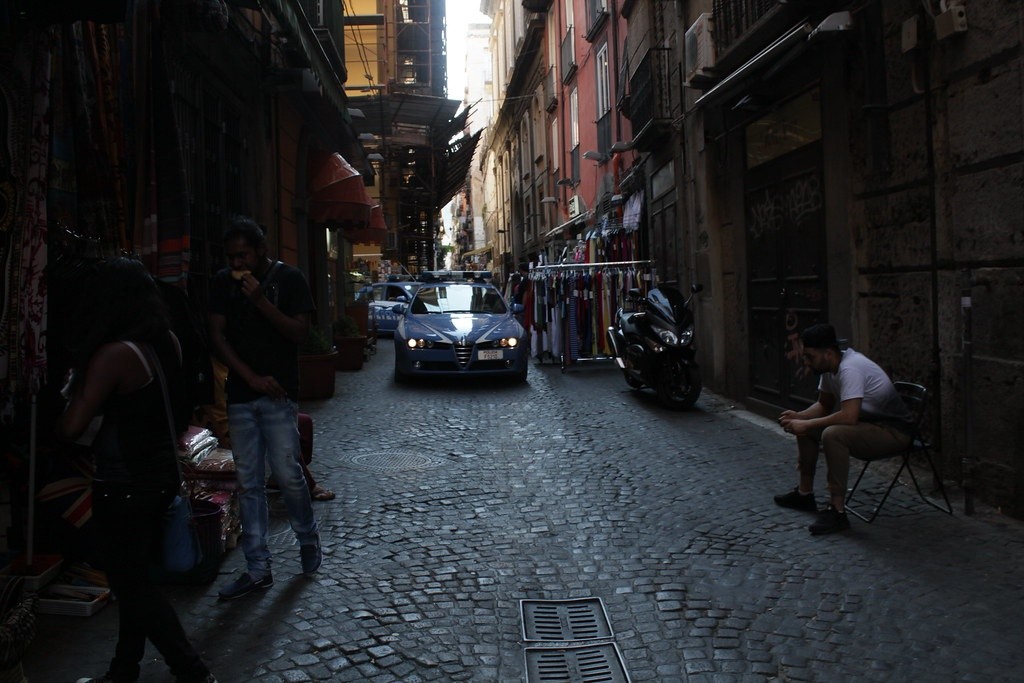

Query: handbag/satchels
<box><xmin>157</xmin><ymin>496</ymin><xmax>203</xmax><ymax>567</ymax></box>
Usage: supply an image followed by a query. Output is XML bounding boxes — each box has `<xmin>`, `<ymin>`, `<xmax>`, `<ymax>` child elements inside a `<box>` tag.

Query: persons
<box><xmin>210</xmin><ymin>219</ymin><xmax>321</xmax><ymax>600</ymax></box>
<box><xmin>512</xmin><ymin>262</ymin><xmax>528</xmax><ymax>294</ymax></box>
<box><xmin>774</xmin><ymin>323</ymin><xmax>917</xmax><ymax>536</ymax></box>
<box><xmin>56</xmin><ymin>257</ymin><xmax>220</xmax><ymax>683</ymax></box>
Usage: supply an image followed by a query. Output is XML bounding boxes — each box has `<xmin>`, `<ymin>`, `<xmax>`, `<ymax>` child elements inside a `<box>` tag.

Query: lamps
<box><xmin>808</xmin><ymin>10</ymin><xmax>858</xmax><ymax>41</ymax></box>
<box><xmin>540</xmin><ymin>197</ymin><xmax>561</xmax><ymax>206</ymax></box>
<box><xmin>557</xmin><ymin>177</ymin><xmax>576</xmax><ymax>188</ymax></box>
<box><xmin>582</xmin><ymin>152</ymin><xmax>608</xmax><ymax>164</ymax></box>
<box><xmin>496</xmin><ymin>230</ymin><xmax>509</xmax><ymax>233</ymax></box>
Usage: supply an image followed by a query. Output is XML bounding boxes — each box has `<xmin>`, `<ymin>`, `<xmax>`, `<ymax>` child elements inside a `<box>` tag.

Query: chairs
<box><xmin>844</xmin><ymin>381</ymin><xmax>953</xmax><ymax>524</ymax></box>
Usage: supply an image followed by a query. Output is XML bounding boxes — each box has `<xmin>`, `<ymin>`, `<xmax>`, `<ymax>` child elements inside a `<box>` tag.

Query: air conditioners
<box><xmin>685</xmin><ymin>13</ymin><xmax>720</xmax><ymax>84</ymax></box>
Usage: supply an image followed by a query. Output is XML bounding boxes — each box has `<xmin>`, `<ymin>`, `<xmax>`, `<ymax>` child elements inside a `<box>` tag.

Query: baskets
<box><xmin>0</xmin><ymin>557</ymin><xmax>64</xmax><ymax>590</ymax></box>
<box><xmin>23</xmin><ymin>584</ymin><xmax>110</xmax><ymax>616</ymax></box>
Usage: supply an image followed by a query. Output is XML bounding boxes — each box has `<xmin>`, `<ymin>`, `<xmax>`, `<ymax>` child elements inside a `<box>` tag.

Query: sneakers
<box><xmin>809</xmin><ymin>505</ymin><xmax>850</xmax><ymax>533</ymax></box>
<box><xmin>774</xmin><ymin>486</ymin><xmax>816</xmax><ymax>511</ymax></box>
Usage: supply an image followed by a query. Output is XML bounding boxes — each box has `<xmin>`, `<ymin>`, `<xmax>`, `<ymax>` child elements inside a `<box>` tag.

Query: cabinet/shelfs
<box><xmin>348</xmin><ymin>280</ymin><xmax>378</xmax><ymax>361</ymax></box>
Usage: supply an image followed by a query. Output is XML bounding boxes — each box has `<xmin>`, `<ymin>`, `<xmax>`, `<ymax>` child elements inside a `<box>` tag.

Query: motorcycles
<box><xmin>605</xmin><ymin>284</ymin><xmax>707</xmax><ymax>407</ymax></box>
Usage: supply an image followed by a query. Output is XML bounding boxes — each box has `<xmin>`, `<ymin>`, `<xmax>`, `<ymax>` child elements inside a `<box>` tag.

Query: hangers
<box><xmin>530</xmin><ymin>263</ymin><xmax>640</xmax><ymax>280</ymax></box>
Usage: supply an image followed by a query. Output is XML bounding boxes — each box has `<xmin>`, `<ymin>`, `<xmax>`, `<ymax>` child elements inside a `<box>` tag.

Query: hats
<box><xmin>801</xmin><ymin>324</ymin><xmax>849</xmax><ymax>347</ymax></box>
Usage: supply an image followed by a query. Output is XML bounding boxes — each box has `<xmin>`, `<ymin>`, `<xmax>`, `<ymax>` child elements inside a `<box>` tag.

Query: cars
<box><xmin>393</xmin><ymin>269</ymin><xmax>530</xmax><ymax>386</ymax></box>
<box><xmin>354</xmin><ymin>282</ymin><xmax>422</xmax><ymax>337</ymax></box>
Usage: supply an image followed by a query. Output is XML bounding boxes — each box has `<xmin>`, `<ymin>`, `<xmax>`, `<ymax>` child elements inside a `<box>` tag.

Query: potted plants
<box><xmin>334</xmin><ymin>315</ymin><xmax>367</xmax><ymax>372</ymax></box>
<box><xmin>178</xmin><ymin>440</ymin><xmax>226</xmax><ymax>586</ymax></box>
<box><xmin>299</xmin><ymin>323</ymin><xmax>340</xmax><ymax>400</ymax></box>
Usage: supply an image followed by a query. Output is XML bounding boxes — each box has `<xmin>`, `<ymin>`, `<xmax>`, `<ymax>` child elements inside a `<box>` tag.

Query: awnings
<box><xmin>307</xmin><ymin>149</ymin><xmax>388</xmax><ymax>244</ymax></box>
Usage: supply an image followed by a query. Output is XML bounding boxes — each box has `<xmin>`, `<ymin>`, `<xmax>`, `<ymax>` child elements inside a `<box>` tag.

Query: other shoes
<box><xmin>218</xmin><ymin>572</ymin><xmax>273</xmax><ymax>600</ymax></box>
<box><xmin>76</xmin><ymin>673</ymin><xmax>138</xmax><ymax>683</ymax></box>
<box><xmin>300</xmin><ymin>533</ymin><xmax>322</xmax><ymax>575</ymax></box>
<box><xmin>312</xmin><ymin>488</ymin><xmax>335</xmax><ymax>500</ymax></box>
